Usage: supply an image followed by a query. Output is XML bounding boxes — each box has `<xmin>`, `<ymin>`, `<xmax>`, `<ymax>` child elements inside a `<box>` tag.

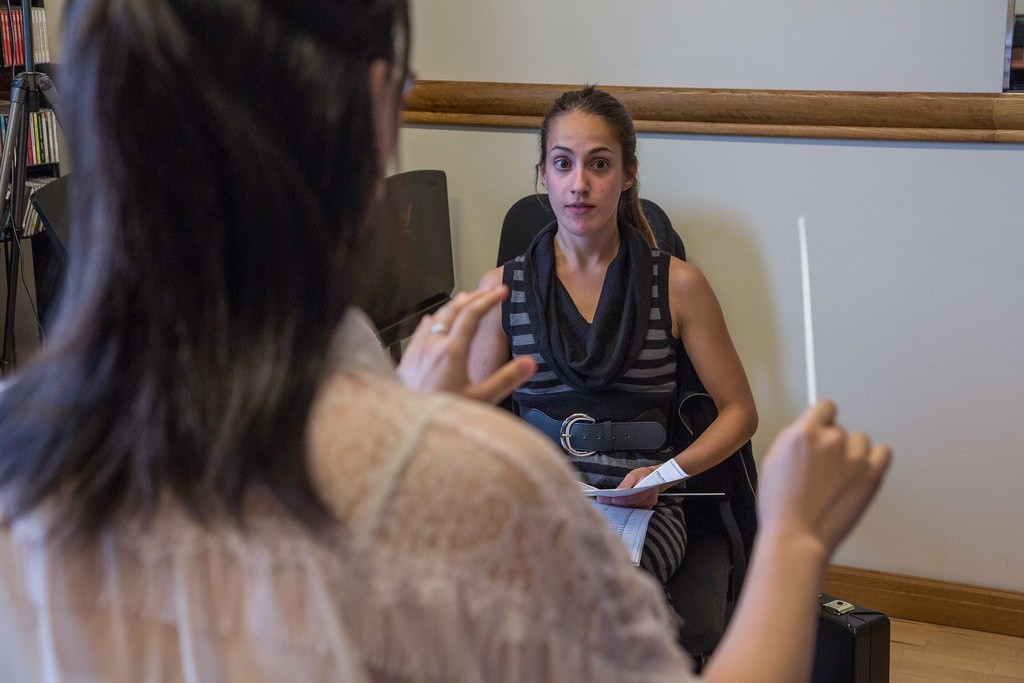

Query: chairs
<box><xmin>366</xmin><ymin>168</ymin><xmax>456</xmax><ymax>369</ymax></box>
<box><xmin>497</xmin><ymin>194</ymin><xmax>732</xmax><ymax>676</ymax></box>
<box><xmin>31</xmin><ymin>170</ymin><xmax>69</xmax><ymax>341</ymax></box>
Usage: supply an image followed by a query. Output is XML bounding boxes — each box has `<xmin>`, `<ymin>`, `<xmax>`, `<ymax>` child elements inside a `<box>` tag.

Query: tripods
<box><xmin>0</xmin><ymin>0</ymin><xmax>75</xmax><ymax>377</ymax></box>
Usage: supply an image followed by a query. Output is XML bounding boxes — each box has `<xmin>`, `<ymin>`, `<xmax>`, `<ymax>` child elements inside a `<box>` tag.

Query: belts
<box><xmin>507</xmin><ymin>406</ymin><xmax>668</xmax><ymax>459</ymax></box>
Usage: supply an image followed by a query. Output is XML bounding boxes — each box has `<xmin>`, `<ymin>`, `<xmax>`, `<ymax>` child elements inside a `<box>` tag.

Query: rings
<box><xmin>430</xmin><ymin>324</ymin><xmax>449</xmax><ymax>333</ymax></box>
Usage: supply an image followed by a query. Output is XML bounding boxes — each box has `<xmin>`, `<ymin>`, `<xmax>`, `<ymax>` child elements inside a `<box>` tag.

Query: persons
<box><xmin>466</xmin><ymin>83</ymin><xmax>759</xmax><ymax>642</ymax></box>
<box><xmin>0</xmin><ymin>0</ymin><xmax>894</xmax><ymax>683</ymax></box>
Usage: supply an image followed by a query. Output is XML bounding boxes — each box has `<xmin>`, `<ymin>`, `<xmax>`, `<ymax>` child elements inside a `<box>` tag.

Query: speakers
<box><xmin>357</xmin><ymin>169</ymin><xmax>455</xmax><ymax>323</ymax></box>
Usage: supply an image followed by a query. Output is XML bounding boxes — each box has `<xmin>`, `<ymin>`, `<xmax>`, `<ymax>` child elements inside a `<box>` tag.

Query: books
<box><xmin>0</xmin><ymin>7</ymin><xmax>59</xmax><ymax>237</ymax></box>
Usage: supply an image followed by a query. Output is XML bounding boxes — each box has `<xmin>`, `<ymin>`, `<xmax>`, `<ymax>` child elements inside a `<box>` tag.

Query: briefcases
<box><xmin>809</xmin><ymin>591</ymin><xmax>892</xmax><ymax>683</ymax></box>
